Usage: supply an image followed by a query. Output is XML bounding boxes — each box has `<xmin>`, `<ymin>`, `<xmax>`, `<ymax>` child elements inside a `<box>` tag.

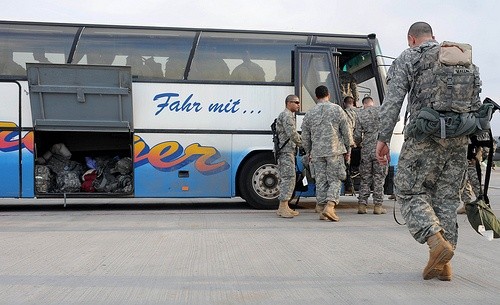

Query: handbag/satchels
<box><xmin>465</xmin><ymin>130</ymin><xmax>500</xmax><ymax>241</ymax></box>
<box><xmin>293</xmin><ymin>164</ymin><xmax>309</xmax><ymax>193</ymax></box>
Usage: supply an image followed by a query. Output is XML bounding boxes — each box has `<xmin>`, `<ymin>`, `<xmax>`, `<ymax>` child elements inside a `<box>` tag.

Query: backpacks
<box><xmin>408</xmin><ymin>41</ymin><xmax>476</xmax><ymax>150</ymax></box>
<box><xmin>35</xmin><ymin>142</ymin><xmax>133</xmax><ymax>194</ymax></box>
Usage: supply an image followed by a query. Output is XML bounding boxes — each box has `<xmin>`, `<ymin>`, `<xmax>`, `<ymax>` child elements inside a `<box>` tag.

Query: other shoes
<box><xmin>457</xmin><ymin>203</ymin><xmax>468</xmax><ymax>214</ymax></box>
<box><xmin>388</xmin><ymin>194</ymin><xmax>395</xmax><ymax>199</ymax></box>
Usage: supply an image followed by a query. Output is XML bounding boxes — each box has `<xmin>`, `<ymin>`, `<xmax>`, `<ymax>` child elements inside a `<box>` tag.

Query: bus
<box><xmin>0</xmin><ymin>20</ymin><xmax>397</xmax><ymax>210</ymax></box>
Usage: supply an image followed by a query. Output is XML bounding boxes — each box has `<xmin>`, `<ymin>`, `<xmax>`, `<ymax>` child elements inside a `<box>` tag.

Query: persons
<box><xmin>277</xmin><ymin>94</ymin><xmax>305</xmax><ymax>218</ymax></box>
<box><xmin>343</xmin><ymin>94</ymin><xmax>387</xmax><ymax>214</ymax></box>
<box><xmin>33</xmin><ymin>48</ymin><xmax>50</xmax><ymax>63</ymax></box>
<box><xmin>300</xmin><ymin>85</ymin><xmax>354</xmax><ymax>222</ymax></box>
<box><xmin>230</xmin><ymin>51</ymin><xmax>265</xmax><ymax>81</ymax></box>
<box><xmin>375</xmin><ymin>21</ymin><xmax>490</xmax><ymax>280</ymax></box>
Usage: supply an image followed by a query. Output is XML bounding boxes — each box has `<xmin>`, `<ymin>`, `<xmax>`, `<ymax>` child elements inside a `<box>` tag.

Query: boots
<box><xmin>319</xmin><ymin>201</ymin><xmax>340</xmax><ymax>221</ymax></box>
<box><xmin>374</xmin><ymin>204</ymin><xmax>387</xmax><ymax>214</ymax></box>
<box><xmin>358</xmin><ymin>204</ymin><xmax>367</xmax><ymax>214</ymax></box>
<box><xmin>423</xmin><ymin>233</ymin><xmax>454</xmax><ymax>282</ymax></box>
<box><xmin>277</xmin><ymin>200</ymin><xmax>300</xmax><ymax>218</ymax></box>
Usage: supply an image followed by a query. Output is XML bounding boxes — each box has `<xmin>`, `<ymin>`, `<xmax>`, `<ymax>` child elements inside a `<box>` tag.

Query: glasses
<box><xmin>290</xmin><ymin>101</ymin><xmax>300</xmax><ymax>104</ymax></box>
